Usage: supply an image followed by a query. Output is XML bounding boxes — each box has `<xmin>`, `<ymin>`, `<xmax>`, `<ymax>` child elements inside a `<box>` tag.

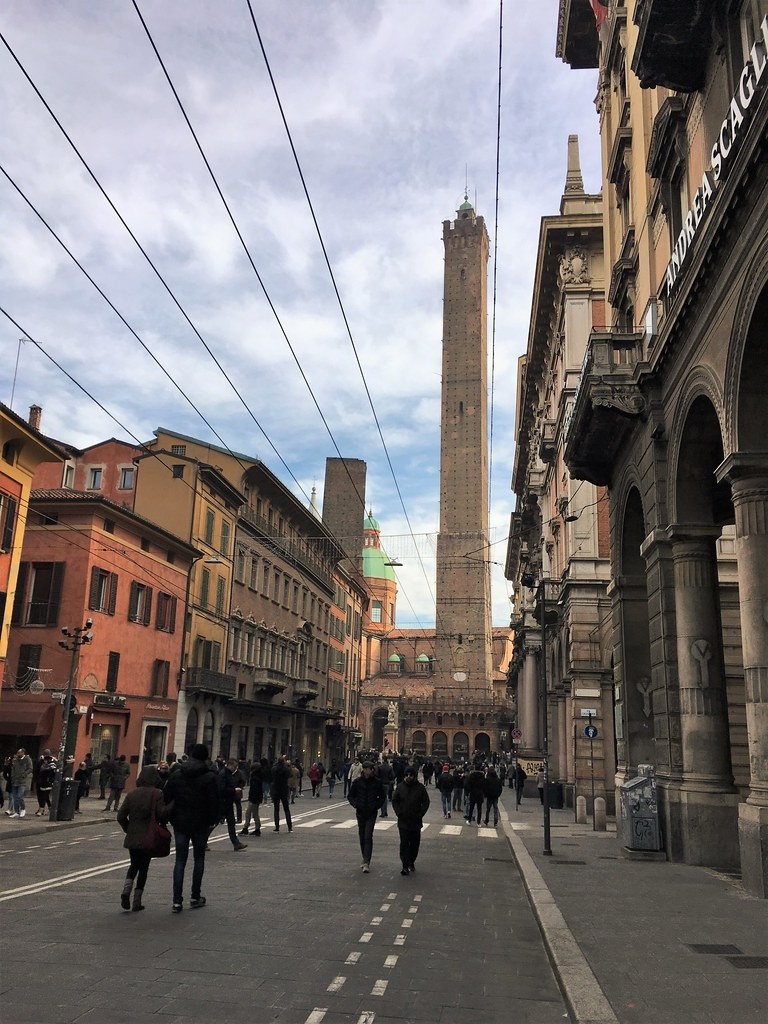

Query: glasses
<box><xmin>404</xmin><ymin>773</ymin><xmax>413</xmax><ymax>777</ymax></box>
<box><xmin>368</xmin><ymin>767</ymin><xmax>375</xmax><ymax>770</ymax></box>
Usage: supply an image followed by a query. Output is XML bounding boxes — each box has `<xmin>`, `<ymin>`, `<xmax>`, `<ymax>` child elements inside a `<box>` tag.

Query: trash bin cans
<box><xmin>545</xmin><ymin>783</ymin><xmax>563</xmax><ymax>809</ymax></box>
<box><xmin>52</xmin><ymin>781</ymin><xmax>81</xmax><ymax>820</ymax></box>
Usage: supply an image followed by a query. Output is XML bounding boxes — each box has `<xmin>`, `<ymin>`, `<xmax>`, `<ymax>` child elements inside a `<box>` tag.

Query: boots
<box><xmin>121</xmin><ymin>878</ymin><xmax>133</xmax><ymax>910</ymax></box>
<box><xmin>132</xmin><ymin>887</ymin><xmax>145</xmax><ymax>911</ymax></box>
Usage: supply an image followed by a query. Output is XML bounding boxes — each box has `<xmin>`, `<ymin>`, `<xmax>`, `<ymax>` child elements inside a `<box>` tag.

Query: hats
<box><xmin>362</xmin><ymin>761</ymin><xmax>375</xmax><ymax>767</ymax></box>
<box><xmin>404</xmin><ymin>768</ymin><xmax>418</xmax><ymax>774</ymax></box>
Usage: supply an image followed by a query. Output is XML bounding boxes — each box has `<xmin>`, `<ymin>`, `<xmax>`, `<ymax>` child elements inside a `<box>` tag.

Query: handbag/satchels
<box><xmin>143</xmin><ymin>822</ymin><xmax>172</xmax><ymax>858</ymax></box>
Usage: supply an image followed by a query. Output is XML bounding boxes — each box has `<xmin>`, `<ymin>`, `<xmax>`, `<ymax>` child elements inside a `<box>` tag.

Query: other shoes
<box><xmin>103</xmin><ymin>808</ymin><xmax>111</xmax><ymax>812</ymax></box>
<box><xmin>98</xmin><ymin>796</ymin><xmax>105</xmax><ymax>800</ymax></box>
<box><xmin>6</xmin><ymin>809</ymin><xmax>11</xmax><ymax>815</ymax></box>
<box><xmin>465</xmin><ymin>821</ymin><xmax>470</xmax><ymax>824</ymax></box>
<box><xmin>37</xmin><ymin>808</ymin><xmax>45</xmax><ymax>815</ymax></box>
<box><xmin>272</xmin><ymin>829</ymin><xmax>279</xmax><ymax>832</ymax></box>
<box><xmin>401</xmin><ymin>865</ymin><xmax>414</xmax><ymax>875</ymax></box>
<box><xmin>477</xmin><ymin>823</ymin><xmax>481</xmax><ymax>828</ymax></box>
<box><xmin>362</xmin><ymin>863</ymin><xmax>369</xmax><ymax>872</ymax></box>
<box><xmin>249</xmin><ymin>830</ymin><xmax>260</xmax><ymax>836</ymax></box>
<box><xmin>234</xmin><ymin>843</ymin><xmax>247</xmax><ymax>850</ymax></box>
<box><xmin>75</xmin><ymin>809</ymin><xmax>82</xmax><ymax>814</ymax></box>
<box><xmin>113</xmin><ymin>809</ymin><xmax>120</xmax><ymax>812</ymax></box>
<box><xmin>288</xmin><ymin>828</ymin><xmax>293</xmax><ymax>832</ymax></box>
<box><xmin>238</xmin><ymin>830</ymin><xmax>248</xmax><ymax>837</ymax></box>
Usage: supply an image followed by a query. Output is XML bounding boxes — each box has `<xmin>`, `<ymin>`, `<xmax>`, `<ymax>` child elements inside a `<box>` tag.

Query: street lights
<box><xmin>520</xmin><ymin>574</ymin><xmax>554</xmax><ymax>855</ymax></box>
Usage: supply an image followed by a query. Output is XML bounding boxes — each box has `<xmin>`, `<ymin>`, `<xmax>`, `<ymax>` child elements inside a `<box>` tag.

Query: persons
<box><xmin>211</xmin><ymin>742</ymin><xmax>515</xmax><ymax>820</ymax></box>
<box><xmin>534</xmin><ymin>768</ymin><xmax>546</xmax><ymax>805</ymax></box>
<box><xmin>161</xmin><ymin>744</ymin><xmax>225</xmax><ymax>914</ymax></box>
<box><xmin>88</xmin><ymin>753</ymin><xmax>112</xmax><ymax>799</ymax></box>
<box><xmin>0</xmin><ymin>767</ymin><xmax>5</xmax><ymax>811</ymax></box>
<box><xmin>463</xmin><ymin>763</ymin><xmax>485</xmax><ymax>828</ymax></box>
<box><xmin>10</xmin><ymin>746</ymin><xmax>34</xmax><ymax>819</ymax></box>
<box><xmin>74</xmin><ymin>761</ymin><xmax>88</xmax><ymax>815</ymax></box>
<box><xmin>201</xmin><ymin>757</ymin><xmax>248</xmax><ymax>852</ymax></box>
<box><xmin>268</xmin><ymin>758</ymin><xmax>296</xmax><ymax>834</ymax></box>
<box><xmin>512</xmin><ymin>763</ymin><xmax>528</xmax><ymax>805</ymax></box>
<box><xmin>115</xmin><ymin>766</ymin><xmax>176</xmax><ymax>912</ymax></box>
<box><xmin>101</xmin><ymin>755</ymin><xmax>131</xmax><ymax>813</ymax></box>
<box><xmin>110</xmin><ymin>757</ymin><xmax>123</xmax><ymax>796</ymax></box>
<box><xmin>346</xmin><ymin>761</ymin><xmax>387</xmax><ymax>873</ymax></box>
<box><xmin>391</xmin><ymin>767</ymin><xmax>431</xmax><ymax>874</ymax></box>
<box><xmin>3</xmin><ymin>753</ymin><xmax>24</xmax><ymax>815</ymax></box>
<box><xmin>35</xmin><ymin>747</ymin><xmax>71</xmax><ymax>817</ymax></box>
<box><xmin>483</xmin><ymin>765</ymin><xmax>503</xmax><ymax>829</ymax></box>
<box><xmin>237</xmin><ymin>762</ymin><xmax>264</xmax><ymax>837</ymax></box>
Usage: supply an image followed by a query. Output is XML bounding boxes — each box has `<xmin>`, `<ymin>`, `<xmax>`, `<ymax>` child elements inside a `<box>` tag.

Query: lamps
<box><xmin>383</xmin><ymin>558</ymin><xmax>403</xmax><ymax>566</ymax></box>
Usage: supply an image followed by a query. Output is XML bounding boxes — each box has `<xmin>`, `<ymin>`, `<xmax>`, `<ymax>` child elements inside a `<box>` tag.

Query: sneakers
<box><xmin>9</xmin><ymin>813</ymin><xmax>19</xmax><ymax>818</ymax></box>
<box><xmin>171</xmin><ymin>896</ymin><xmax>183</xmax><ymax>913</ymax></box>
<box><xmin>190</xmin><ymin>894</ymin><xmax>206</xmax><ymax>907</ymax></box>
<box><xmin>20</xmin><ymin>809</ymin><xmax>26</xmax><ymax>818</ymax></box>
